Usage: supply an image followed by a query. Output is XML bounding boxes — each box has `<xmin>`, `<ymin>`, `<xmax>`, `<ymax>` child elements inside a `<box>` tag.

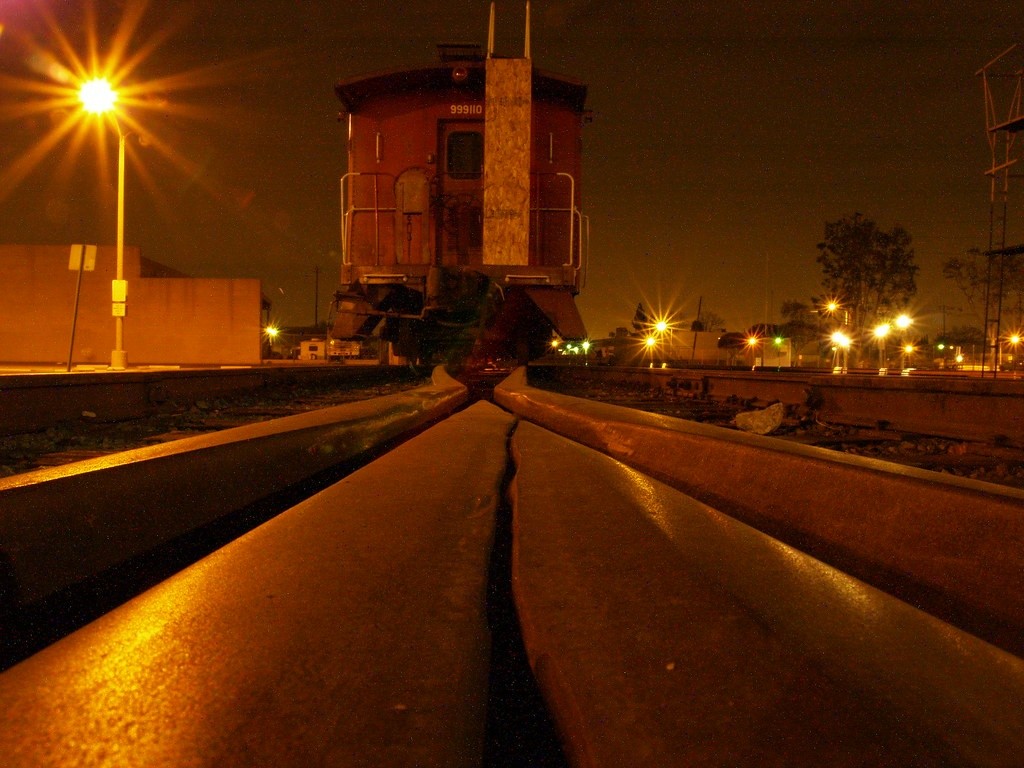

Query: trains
<box><xmin>324</xmin><ymin>0</ymin><xmax>595</xmax><ymax>375</ymax></box>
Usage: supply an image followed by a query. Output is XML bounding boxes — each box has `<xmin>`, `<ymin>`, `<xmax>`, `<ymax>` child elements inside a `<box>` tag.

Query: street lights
<box><xmin>875</xmin><ymin>315</ymin><xmax>915</xmax><ymax>371</ymax></box>
<box><xmin>655</xmin><ymin>320</ymin><xmax>669</xmax><ymax>366</ymax></box>
<box><xmin>831</xmin><ymin>332</ymin><xmax>841</xmax><ymax>373</ymax></box>
<box><xmin>647</xmin><ymin>337</ymin><xmax>657</xmax><ymax>366</ymax></box>
<box><xmin>774</xmin><ymin>337</ymin><xmax>785</xmax><ymax>374</ymax></box>
<box><xmin>748</xmin><ymin>335</ymin><xmax>760</xmax><ymax>373</ymax></box>
<box><xmin>80</xmin><ymin>77</ymin><xmax>127</xmax><ymax>371</ymax></box>
<box><xmin>843</xmin><ymin>335</ymin><xmax>852</xmax><ymax>374</ymax></box>
